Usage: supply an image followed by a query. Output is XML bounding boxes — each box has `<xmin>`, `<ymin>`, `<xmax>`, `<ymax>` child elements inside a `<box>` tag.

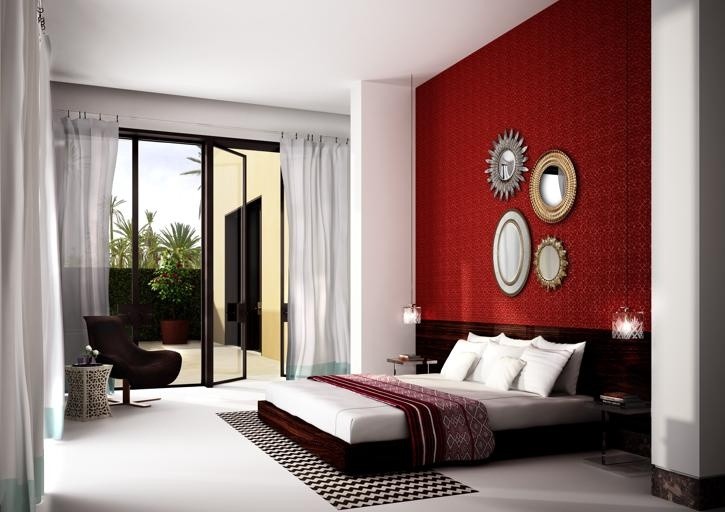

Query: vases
<box><xmin>158</xmin><ymin>318</ymin><xmax>192</xmax><ymax>345</ymax></box>
<box><xmin>65</xmin><ymin>363</ymin><xmax>113</xmax><ymax>422</ymax></box>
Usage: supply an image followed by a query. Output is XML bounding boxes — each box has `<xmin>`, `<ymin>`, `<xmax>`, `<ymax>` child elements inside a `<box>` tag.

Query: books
<box><xmin>600</xmin><ymin>389</ymin><xmax>645</xmax><ymax>409</ymax></box>
<box><xmin>399</xmin><ymin>353</ymin><xmax>424</xmax><ymax>367</ymax></box>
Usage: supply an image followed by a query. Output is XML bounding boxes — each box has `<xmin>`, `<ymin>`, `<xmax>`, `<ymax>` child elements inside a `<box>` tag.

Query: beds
<box><xmin>255</xmin><ymin>374</ymin><xmax>596</xmax><ymax>479</ymax></box>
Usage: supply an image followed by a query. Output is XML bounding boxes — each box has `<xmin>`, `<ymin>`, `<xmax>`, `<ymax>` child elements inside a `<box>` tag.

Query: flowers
<box><xmin>146</xmin><ymin>263</ymin><xmax>198</xmax><ymax>318</ymax></box>
<box><xmin>79</xmin><ymin>343</ymin><xmax>103</xmax><ymax>365</ymax></box>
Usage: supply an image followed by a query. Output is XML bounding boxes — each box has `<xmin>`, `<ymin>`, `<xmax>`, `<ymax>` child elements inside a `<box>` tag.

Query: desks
<box><xmin>585</xmin><ymin>400</ymin><xmax>650</xmax><ymax>466</ymax></box>
<box><xmin>386</xmin><ymin>354</ymin><xmax>437</xmax><ymax>375</ymax></box>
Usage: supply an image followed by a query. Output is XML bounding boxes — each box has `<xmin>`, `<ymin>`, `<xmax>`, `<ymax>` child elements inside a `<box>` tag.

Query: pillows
<box><xmin>441</xmin><ymin>332</ymin><xmax>586</xmax><ymax>398</ymax></box>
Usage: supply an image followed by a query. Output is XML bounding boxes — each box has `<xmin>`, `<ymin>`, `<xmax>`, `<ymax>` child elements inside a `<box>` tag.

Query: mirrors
<box><xmin>529</xmin><ymin>150</ymin><xmax>577</xmax><ymax>224</ymax></box>
<box><xmin>492</xmin><ymin>209</ymin><xmax>531</xmax><ymax>296</ymax></box>
<box><xmin>485</xmin><ymin>129</ymin><xmax>529</xmax><ymax>201</ymax></box>
<box><xmin>534</xmin><ymin>234</ymin><xmax>568</xmax><ymax>291</ymax></box>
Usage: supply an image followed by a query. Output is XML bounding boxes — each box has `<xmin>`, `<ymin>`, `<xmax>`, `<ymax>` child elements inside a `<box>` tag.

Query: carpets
<box><xmin>215</xmin><ymin>409</ymin><xmax>480</xmax><ymax>511</ymax></box>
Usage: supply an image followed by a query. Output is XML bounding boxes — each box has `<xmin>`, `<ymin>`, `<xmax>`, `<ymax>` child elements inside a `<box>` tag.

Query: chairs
<box><xmin>82</xmin><ymin>314</ymin><xmax>183</xmax><ymax>408</ymax></box>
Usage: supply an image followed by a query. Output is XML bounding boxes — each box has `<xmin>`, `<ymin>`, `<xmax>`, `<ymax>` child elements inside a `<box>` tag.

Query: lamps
<box><xmin>611</xmin><ymin>2</ymin><xmax>645</xmax><ymax>341</ymax></box>
<box><xmin>399</xmin><ymin>73</ymin><xmax>422</xmax><ymax>326</ymax></box>
